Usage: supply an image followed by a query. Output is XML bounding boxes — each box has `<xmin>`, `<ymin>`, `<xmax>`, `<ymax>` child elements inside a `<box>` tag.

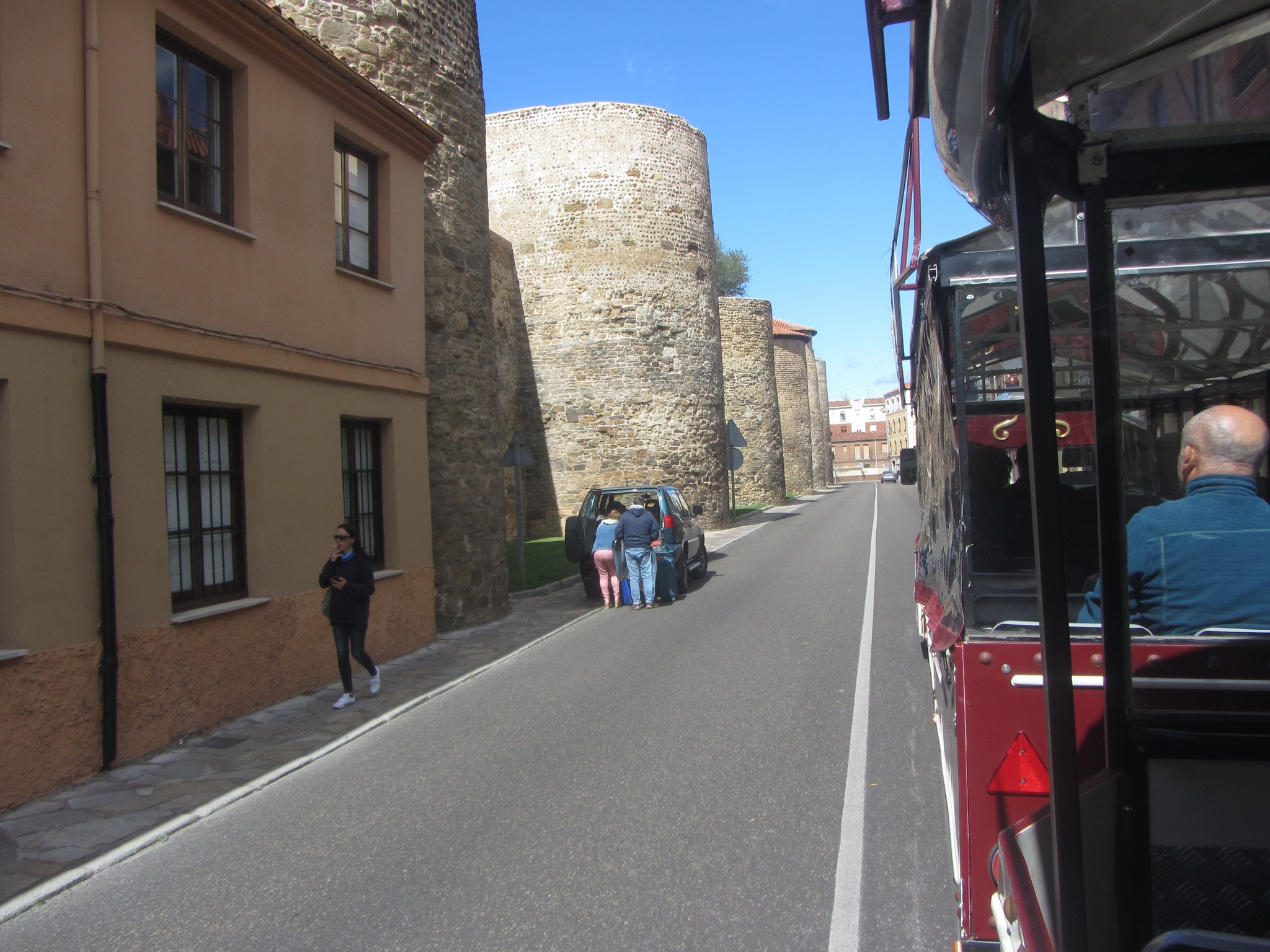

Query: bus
<box><xmin>865</xmin><ymin>0</ymin><xmax>1270</xmax><ymax>952</ymax></box>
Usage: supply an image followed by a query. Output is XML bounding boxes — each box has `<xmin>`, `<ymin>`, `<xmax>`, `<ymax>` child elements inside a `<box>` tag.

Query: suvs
<box><xmin>575</xmin><ymin>484</ymin><xmax>709</xmax><ymax>601</ymax></box>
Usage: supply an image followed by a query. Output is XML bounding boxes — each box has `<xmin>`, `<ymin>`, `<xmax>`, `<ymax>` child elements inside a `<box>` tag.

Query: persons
<box><xmin>1077</xmin><ymin>404</ymin><xmax>1270</xmax><ymax>636</ymax></box>
<box><xmin>591</xmin><ymin>509</ymin><xmax>622</xmax><ymax>609</ymax></box>
<box><xmin>319</xmin><ymin>523</ymin><xmax>381</xmax><ymax>709</ymax></box>
<box><xmin>968</xmin><ymin>442</ymin><xmax>1079</xmax><ymax>555</ymax></box>
<box><xmin>614</xmin><ymin>496</ymin><xmax>659</xmax><ymax>610</ymax></box>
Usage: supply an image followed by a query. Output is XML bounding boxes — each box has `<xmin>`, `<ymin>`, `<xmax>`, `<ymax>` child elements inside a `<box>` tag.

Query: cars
<box><xmin>880</xmin><ymin>471</ymin><xmax>897</xmax><ymax>483</ymax></box>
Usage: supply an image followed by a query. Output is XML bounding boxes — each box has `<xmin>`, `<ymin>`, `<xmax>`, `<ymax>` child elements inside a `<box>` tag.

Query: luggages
<box><xmin>656</xmin><ymin>556</ymin><xmax>679</xmax><ymax>603</ymax></box>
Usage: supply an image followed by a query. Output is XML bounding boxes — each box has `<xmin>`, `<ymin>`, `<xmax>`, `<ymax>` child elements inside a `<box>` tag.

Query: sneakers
<box><xmin>368</xmin><ymin>665</ymin><xmax>382</xmax><ymax>695</ymax></box>
<box><xmin>333</xmin><ymin>693</ymin><xmax>356</xmax><ymax>709</ymax></box>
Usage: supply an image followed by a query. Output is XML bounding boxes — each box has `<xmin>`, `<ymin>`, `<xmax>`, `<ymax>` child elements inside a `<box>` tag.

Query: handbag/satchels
<box><xmin>620</xmin><ymin>578</ymin><xmax>633</xmax><ymax>606</ymax></box>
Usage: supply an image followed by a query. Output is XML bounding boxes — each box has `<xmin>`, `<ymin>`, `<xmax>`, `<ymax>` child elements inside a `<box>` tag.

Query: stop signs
<box><xmin>884</xmin><ymin>461</ymin><xmax>888</xmax><ymax>466</ymax></box>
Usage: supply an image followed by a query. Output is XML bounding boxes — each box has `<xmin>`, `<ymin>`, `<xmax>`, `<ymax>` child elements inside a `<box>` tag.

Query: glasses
<box><xmin>334</xmin><ymin>534</ymin><xmax>352</xmax><ymax>541</ymax></box>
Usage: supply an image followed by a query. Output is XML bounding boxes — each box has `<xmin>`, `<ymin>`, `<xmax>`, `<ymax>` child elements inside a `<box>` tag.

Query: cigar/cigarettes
<box><xmin>334</xmin><ymin>545</ymin><xmax>337</xmax><ymax>547</ymax></box>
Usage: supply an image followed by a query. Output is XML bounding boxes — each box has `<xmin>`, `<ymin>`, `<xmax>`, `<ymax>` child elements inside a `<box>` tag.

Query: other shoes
<box><xmin>614</xmin><ymin>601</ymin><xmax>622</xmax><ymax>608</ymax></box>
<box><xmin>645</xmin><ymin>601</ymin><xmax>660</xmax><ymax>609</ymax></box>
<box><xmin>630</xmin><ymin>604</ymin><xmax>641</xmax><ymax>611</ymax></box>
<box><xmin>604</xmin><ymin>602</ymin><xmax>610</xmax><ymax>609</ymax></box>
